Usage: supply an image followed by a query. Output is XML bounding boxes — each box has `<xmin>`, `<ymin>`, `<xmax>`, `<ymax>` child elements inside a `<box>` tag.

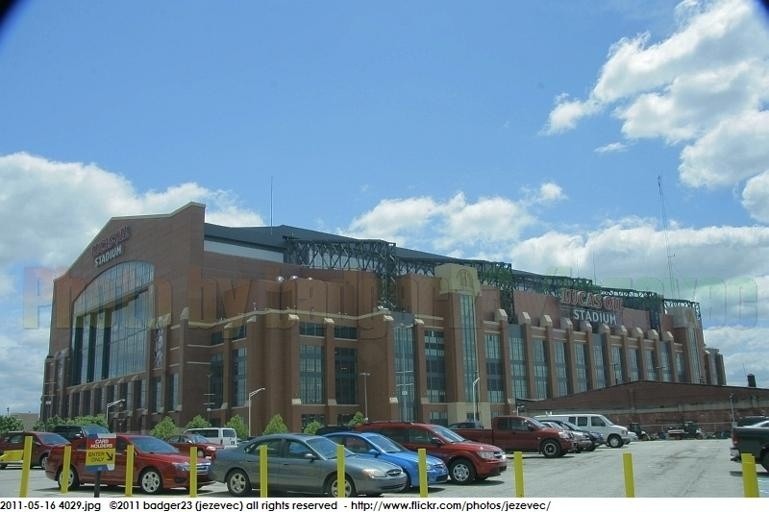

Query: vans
<box><xmin>53</xmin><ymin>425</ymin><xmax>108</xmax><ymax>442</ymax></box>
<box><xmin>534</xmin><ymin>415</ymin><xmax>630</xmax><ymax>448</ymax></box>
<box><xmin>183</xmin><ymin>427</ymin><xmax>237</xmax><ymax>447</ymax></box>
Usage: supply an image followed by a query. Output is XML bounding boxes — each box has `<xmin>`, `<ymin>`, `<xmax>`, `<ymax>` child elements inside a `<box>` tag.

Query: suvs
<box><xmin>354</xmin><ymin>422</ymin><xmax>506</xmax><ymax>484</ymax></box>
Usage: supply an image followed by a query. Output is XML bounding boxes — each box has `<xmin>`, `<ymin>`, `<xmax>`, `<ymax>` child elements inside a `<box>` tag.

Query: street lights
<box><xmin>248</xmin><ymin>388</ymin><xmax>265</xmax><ymax>436</ymax></box>
<box><xmin>472</xmin><ymin>378</ymin><xmax>482</xmax><ymax>422</ymax></box>
<box><xmin>107</xmin><ymin>399</ymin><xmax>126</xmax><ymax>427</ymax></box>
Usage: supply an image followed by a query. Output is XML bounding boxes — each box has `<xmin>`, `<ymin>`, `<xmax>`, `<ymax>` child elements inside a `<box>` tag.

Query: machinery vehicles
<box><xmin>668</xmin><ymin>423</ymin><xmax>704</xmax><ymax>440</ymax></box>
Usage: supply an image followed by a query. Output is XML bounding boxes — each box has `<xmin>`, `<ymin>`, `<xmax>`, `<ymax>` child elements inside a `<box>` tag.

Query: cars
<box><xmin>47</xmin><ymin>434</ymin><xmax>215</xmax><ymax>493</ymax></box>
<box><xmin>0</xmin><ymin>431</ymin><xmax>72</xmax><ymax>469</ymax></box>
<box><xmin>624</xmin><ymin>432</ymin><xmax>638</xmax><ymax>444</ymax></box>
<box><xmin>540</xmin><ymin>419</ymin><xmax>603</xmax><ymax>452</ymax></box>
<box><xmin>323</xmin><ymin>432</ymin><xmax>448</xmax><ymax>492</ymax></box>
<box><xmin>208</xmin><ymin>433</ymin><xmax>404</xmax><ymax>497</ymax></box>
<box><xmin>730</xmin><ymin>421</ymin><xmax>769</xmax><ymax>471</ymax></box>
<box><xmin>163</xmin><ymin>434</ymin><xmax>224</xmax><ymax>457</ymax></box>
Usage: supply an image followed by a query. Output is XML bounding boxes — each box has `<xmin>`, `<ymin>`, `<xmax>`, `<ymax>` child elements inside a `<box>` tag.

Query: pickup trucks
<box><xmin>448</xmin><ymin>416</ymin><xmax>577</xmax><ymax>458</ymax></box>
